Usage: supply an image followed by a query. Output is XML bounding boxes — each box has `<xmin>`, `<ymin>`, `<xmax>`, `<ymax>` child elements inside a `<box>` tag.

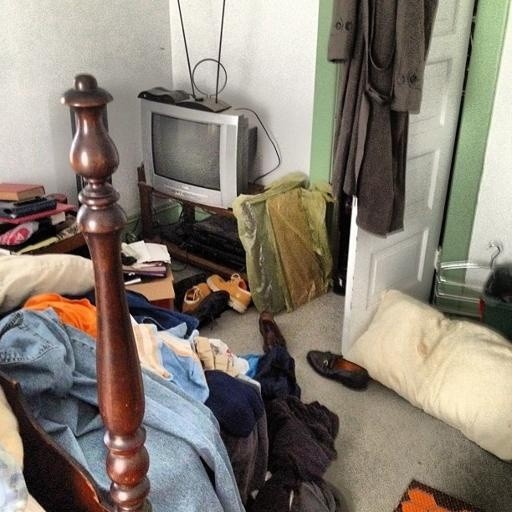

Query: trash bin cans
<box><xmin>479</xmin><ymin>291</ymin><xmax>511</xmax><ymax>344</ymax></box>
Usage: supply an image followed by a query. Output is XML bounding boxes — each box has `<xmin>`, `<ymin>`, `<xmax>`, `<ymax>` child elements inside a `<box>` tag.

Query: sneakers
<box><xmin>181</xmin><ymin>274</ymin><xmax>251</xmax><ymax>330</ymax></box>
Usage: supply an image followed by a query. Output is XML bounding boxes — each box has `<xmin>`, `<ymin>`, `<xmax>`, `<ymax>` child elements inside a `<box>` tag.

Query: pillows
<box><xmin>343</xmin><ymin>287</ymin><xmax>512</xmax><ymax>466</ymax></box>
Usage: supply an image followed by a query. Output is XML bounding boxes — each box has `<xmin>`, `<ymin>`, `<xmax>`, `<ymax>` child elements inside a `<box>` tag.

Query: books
<box><xmin>0</xmin><ymin>183</ymin><xmax>45</xmax><ymax>202</ymax></box>
<box><xmin>121</xmin><ymin>240</ymin><xmax>171</xmax><ymax>278</ymax></box>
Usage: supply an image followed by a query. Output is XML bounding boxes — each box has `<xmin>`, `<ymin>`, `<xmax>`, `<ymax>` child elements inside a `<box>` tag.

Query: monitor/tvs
<box><xmin>140</xmin><ymin>99</ymin><xmax>259</xmax><ymax>211</ymax></box>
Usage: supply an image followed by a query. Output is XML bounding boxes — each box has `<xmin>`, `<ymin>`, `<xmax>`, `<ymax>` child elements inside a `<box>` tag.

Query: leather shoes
<box><xmin>259</xmin><ymin>310</ymin><xmax>282</xmax><ymax>353</ymax></box>
<box><xmin>307</xmin><ymin>349</ymin><xmax>368</xmax><ymax>389</ymax></box>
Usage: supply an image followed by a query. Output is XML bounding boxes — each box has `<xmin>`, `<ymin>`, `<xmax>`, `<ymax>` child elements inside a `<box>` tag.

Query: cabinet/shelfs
<box><xmin>139</xmin><ymin>182</ymin><xmax>266</xmax><ymax>288</ymax></box>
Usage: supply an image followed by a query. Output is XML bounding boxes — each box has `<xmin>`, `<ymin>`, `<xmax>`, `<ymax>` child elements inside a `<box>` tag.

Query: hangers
<box><xmin>437</xmin><ymin>241</ymin><xmax>501</xmax><ymax>292</ymax></box>
